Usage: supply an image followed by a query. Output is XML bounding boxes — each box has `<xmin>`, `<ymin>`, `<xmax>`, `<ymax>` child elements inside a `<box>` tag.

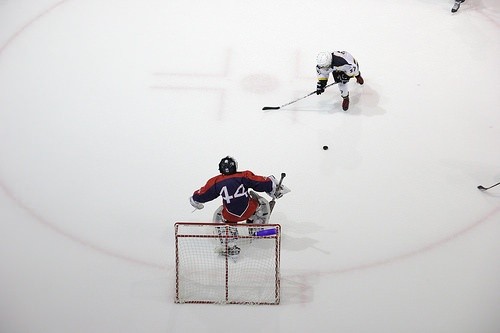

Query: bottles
<box><xmin>252</xmin><ymin>228</ymin><xmax>277</xmax><ymax>236</ymax></box>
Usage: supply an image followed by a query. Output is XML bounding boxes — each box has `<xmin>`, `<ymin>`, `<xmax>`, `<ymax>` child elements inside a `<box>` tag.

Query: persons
<box><xmin>189</xmin><ymin>157</ymin><xmax>284</xmax><ymax>255</ymax></box>
<box><xmin>316</xmin><ymin>51</ymin><xmax>365</xmax><ymax>111</ymax></box>
<box><xmin>451</xmin><ymin>0</ymin><xmax>464</xmax><ymax>13</ymax></box>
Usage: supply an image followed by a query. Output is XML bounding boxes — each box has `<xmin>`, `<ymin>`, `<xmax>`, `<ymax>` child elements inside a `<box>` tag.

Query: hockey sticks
<box><xmin>266</xmin><ymin>173</ymin><xmax>285</xmax><ymax>223</ymax></box>
<box><xmin>477</xmin><ymin>183</ymin><xmax>500</xmax><ymax>192</ymax></box>
<box><xmin>262</xmin><ymin>81</ymin><xmax>341</xmax><ymax>111</ymax></box>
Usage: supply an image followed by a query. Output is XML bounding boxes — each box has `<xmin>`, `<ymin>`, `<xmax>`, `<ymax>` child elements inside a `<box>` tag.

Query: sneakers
<box><xmin>343</xmin><ymin>93</ymin><xmax>349</xmax><ymax>111</ymax></box>
<box><xmin>223</xmin><ymin>247</ymin><xmax>240</xmax><ymax>254</ymax></box>
<box><xmin>249</xmin><ymin>228</ymin><xmax>263</xmax><ymax>234</ymax></box>
<box><xmin>451</xmin><ymin>2</ymin><xmax>460</xmax><ymax>13</ymax></box>
<box><xmin>355</xmin><ymin>72</ymin><xmax>363</xmax><ymax>85</ymax></box>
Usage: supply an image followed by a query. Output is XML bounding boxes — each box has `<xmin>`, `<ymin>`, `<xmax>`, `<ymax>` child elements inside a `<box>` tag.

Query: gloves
<box><xmin>337</xmin><ymin>71</ymin><xmax>350</xmax><ymax>84</ymax></box>
<box><xmin>317</xmin><ymin>80</ymin><xmax>327</xmax><ymax>95</ymax></box>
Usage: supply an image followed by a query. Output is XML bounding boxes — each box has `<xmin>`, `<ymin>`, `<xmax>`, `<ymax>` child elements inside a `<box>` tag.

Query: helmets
<box><xmin>316</xmin><ymin>51</ymin><xmax>332</xmax><ymax>67</ymax></box>
<box><xmin>219</xmin><ymin>157</ymin><xmax>238</xmax><ymax>175</ymax></box>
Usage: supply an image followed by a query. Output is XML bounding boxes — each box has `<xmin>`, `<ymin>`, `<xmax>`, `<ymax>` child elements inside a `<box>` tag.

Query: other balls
<box><xmin>323</xmin><ymin>145</ymin><xmax>328</xmax><ymax>150</ymax></box>
